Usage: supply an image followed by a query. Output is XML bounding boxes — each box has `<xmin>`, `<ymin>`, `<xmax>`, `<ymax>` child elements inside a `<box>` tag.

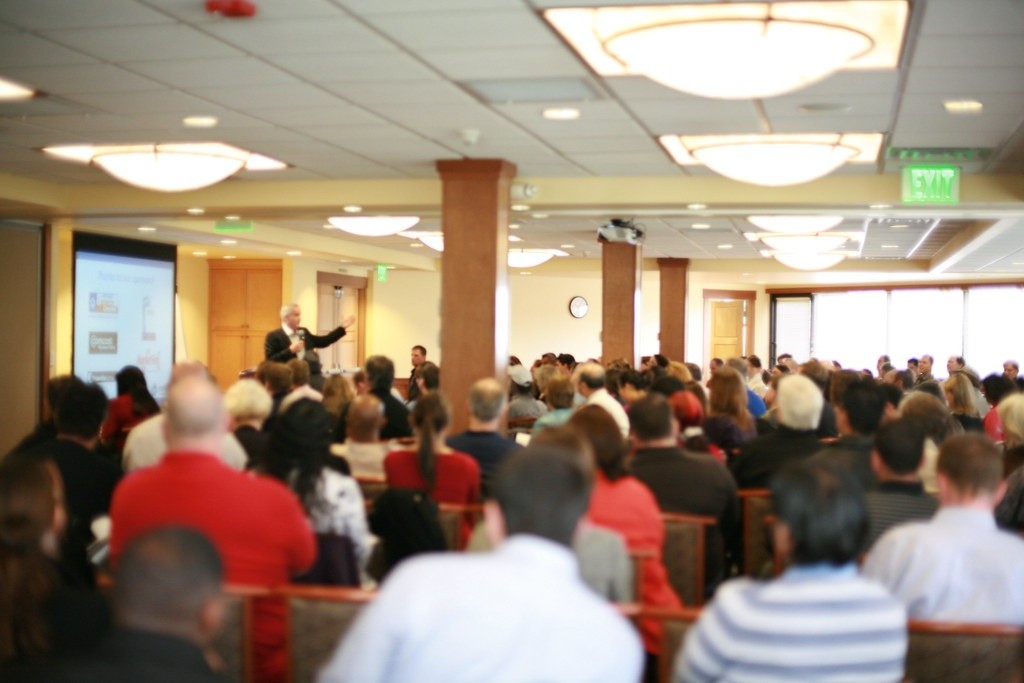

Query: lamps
<box><xmin>600</xmin><ymin>18</ymin><xmax>876</xmax><ymax>270</ymax></box>
<box><xmin>91</xmin><ymin>150</ymin><xmax>556</xmax><ymax>268</ymax></box>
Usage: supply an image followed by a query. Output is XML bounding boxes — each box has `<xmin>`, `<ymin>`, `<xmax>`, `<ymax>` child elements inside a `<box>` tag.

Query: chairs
<box><xmin>645</xmin><ymin>606</ymin><xmax>700</xmax><ymax>681</ymax></box>
<box><xmin>205</xmin><ymin>583</ymin><xmax>271</xmax><ymax>677</ymax></box>
<box><xmin>739</xmin><ymin>489</ymin><xmax>786</xmax><ymax>582</ymax></box>
<box><xmin>358</xmin><ymin>475</ymin><xmax>387</xmax><ymax>511</ymax></box>
<box><xmin>440</xmin><ymin>501</ymin><xmax>464</xmax><ymax>548</ymax></box>
<box><xmin>657</xmin><ymin>515</ymin><xmax>716</xmax><ymax>605</ymax></box>
<box><xmin>900</xmin><ymin>620</ymin><xmax>1022</xmax><ymax>682</ymax></box>
<box><xmin>275</xmin><ymin>583</ymin><xmax>375</xmax><ymax>681</ymax></box>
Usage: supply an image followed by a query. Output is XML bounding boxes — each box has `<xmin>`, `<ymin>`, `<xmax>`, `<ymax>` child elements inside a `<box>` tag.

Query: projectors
<box><xmin>598</xmin><ymin>225</ymin><xmax>645</xmax><ymax>246</ymax></box>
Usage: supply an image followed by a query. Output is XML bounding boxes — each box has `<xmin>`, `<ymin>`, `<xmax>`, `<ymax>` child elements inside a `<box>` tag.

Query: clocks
<box><xmin>570</xmin><ymin>296</ymin><xmax>589</xmax><ymax>318</ymax></box>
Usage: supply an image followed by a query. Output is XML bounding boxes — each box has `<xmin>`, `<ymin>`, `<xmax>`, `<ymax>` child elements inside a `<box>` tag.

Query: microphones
<box><xmin>298</xmin><ymin>330</ymin><xmax>304</xmax><ymax>341</ymax></box>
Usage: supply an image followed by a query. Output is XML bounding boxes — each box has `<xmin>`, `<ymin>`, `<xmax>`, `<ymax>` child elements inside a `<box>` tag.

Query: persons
<box><xmin>0</xmin><ymin>344</ymin><xmax>1024</xmax><ymax>682</ymax></box>
<box><xmin>264</xmin><ymin>303</ymin><xmax>356</xmax><ymax>363</ymax></box>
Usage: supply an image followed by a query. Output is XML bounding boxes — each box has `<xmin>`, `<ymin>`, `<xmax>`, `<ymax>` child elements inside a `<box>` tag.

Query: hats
<box><xmin>775</xmin><ymin>372</ymin><xmax>825</xmax><ymax>431</ymax></box>
<box><xmin>505</xmin><ymin>362</ymin><xmax>534</xmax><ymax>388</ymax></box>
<box><xmin>279</xmin><ymin>396</ymin><xmax>332</xmax><ymax>449</ymax></box>
